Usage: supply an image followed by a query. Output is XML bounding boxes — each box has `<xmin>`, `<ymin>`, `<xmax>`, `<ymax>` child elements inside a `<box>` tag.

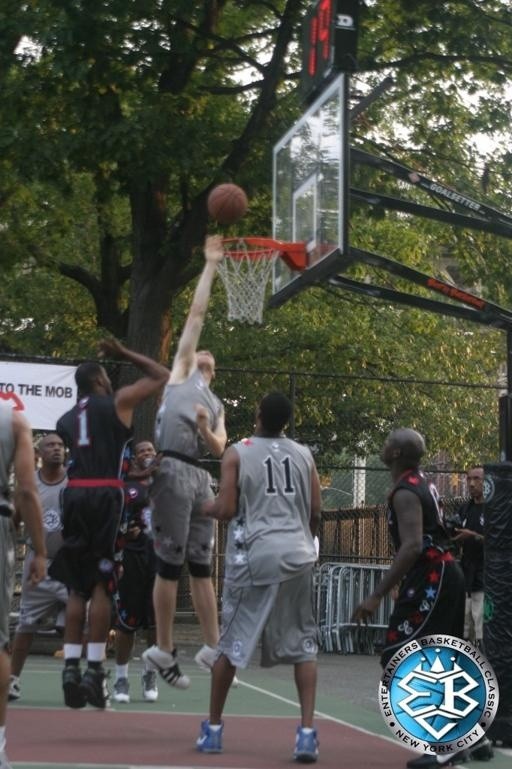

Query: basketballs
<box><xmin>208</xmin><ymin>184</ymin><xmax>249</xmax><ymax>225</ymax></box>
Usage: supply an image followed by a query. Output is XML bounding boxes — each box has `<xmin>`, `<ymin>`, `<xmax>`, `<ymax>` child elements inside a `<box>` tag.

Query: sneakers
<box><xmin>142</xmin><ymin>666</ymin><xmax>158</xmax><ymax>701</ymax></box>
<box><xmin>7</xmin><ymin>674</ymin><xmax>22</xmax><ymax>699</ymax></box>
<box><xmin>196</xmin><ymin>719</ymin><xmax>224</xmax><ymax>753</ymax></box>
<box><xmin>292</xmin><ymin>726</ymin><xmax>319</xmax><ymax>762</ymax></box>
<box><xmin>81</xmin><ymin>667</ymin><xmax>110</xmax><ymax>707</ymax></box>
<box><xmin>194</xmin><ymin>646</ymin><xmax>239</xmax><ymax>688</ymax></box>
<box><xmin>468</xmin><ymin>734</ymin><xmax>494</xmax><ymax>761</ymax></box>
<box><xmin>61</xmin><ymin>665</ymin><xmax>86</xmax><ymax>708</ymax></box>
<box><xmin>115</xmin><ymin>677</ymin><xmax>130</xmax><ymax>704</ymax></box>
<box><xmin>406</xmin><ymin>750</ymin><xmax>467</xmax><ymax>769</ymax></box>
<box><xmin>142</xmin><ymin>647</ymin><xmax>190</xmax><ymax>688</ymax></box>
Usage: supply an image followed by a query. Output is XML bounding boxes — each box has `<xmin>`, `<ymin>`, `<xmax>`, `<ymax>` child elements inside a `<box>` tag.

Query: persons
<box><xmin>197</xmin><ymin>393</ymin><xmax>323</xmax><ymax>763</ymax></box>
<box><xmin>0</xmin><ymin>233</ymin><xmax>227</xmax><ymax>768</ymax></box>
<box><xmin>449</xmin><ymin>465</ymin><xmax>485</xmax><ymax>652</ymax></box>
<box><xmin>349</xmin><ymin>428</ymin><xmax>496</xmax><ymax>769</ymax></box>
